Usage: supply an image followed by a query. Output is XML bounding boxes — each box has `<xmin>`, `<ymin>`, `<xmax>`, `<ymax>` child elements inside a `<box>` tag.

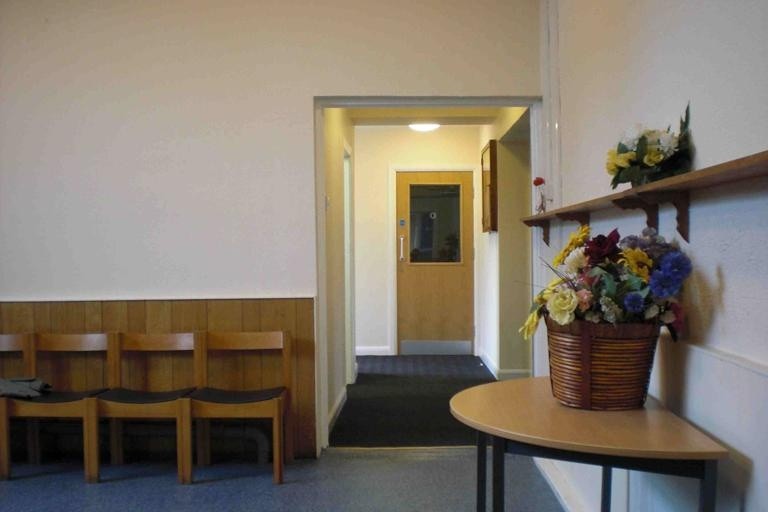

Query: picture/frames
<box><xmin>481</xmin><ymin>139</ymin><xmax>498</xmax><ymax>232</ymax></box>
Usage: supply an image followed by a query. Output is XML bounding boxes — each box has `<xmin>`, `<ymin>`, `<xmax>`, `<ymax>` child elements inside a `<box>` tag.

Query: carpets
<box><xmin>328</xmin><ymin>354</ymin><xmax>497</xmax><ymax>448</ymax></box>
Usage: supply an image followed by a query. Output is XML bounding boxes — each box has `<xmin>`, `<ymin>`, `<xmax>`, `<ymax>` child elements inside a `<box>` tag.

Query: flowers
<box><xmin>533</xmin><ymin>176</ymin><xmax>546</xmax><ymax>215</ymax></box>
<box><xmin>517</xmin><ymin>224</ymin><xmax>693</xmax><ymax>342</ymax></box>
<box><xmin>603</xmin><ymin>99</ymin><xmax>690</xmax><ymax>190</ymax></box>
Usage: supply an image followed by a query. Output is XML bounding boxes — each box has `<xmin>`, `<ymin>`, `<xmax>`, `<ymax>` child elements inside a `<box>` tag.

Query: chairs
<box><xmin>183</xmin><ymin>333</ymin><xmax>292</xmax><ymax>484</ymax></box>
<box><xmin>87</xmin><ymin>331</ymin><xmax>202</xmax><ymax>484</ymax></box>
<box><xmin>2</xmin><ymin>331</ymin><xmax>114</xmax><ymax>484</ymax></box>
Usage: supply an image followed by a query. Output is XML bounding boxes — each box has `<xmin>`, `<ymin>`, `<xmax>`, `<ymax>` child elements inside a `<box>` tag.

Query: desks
<box><xmin>449</xmin><ymin>375</ymin><xmax>729</xmax><ymax>512</ymax></box>
